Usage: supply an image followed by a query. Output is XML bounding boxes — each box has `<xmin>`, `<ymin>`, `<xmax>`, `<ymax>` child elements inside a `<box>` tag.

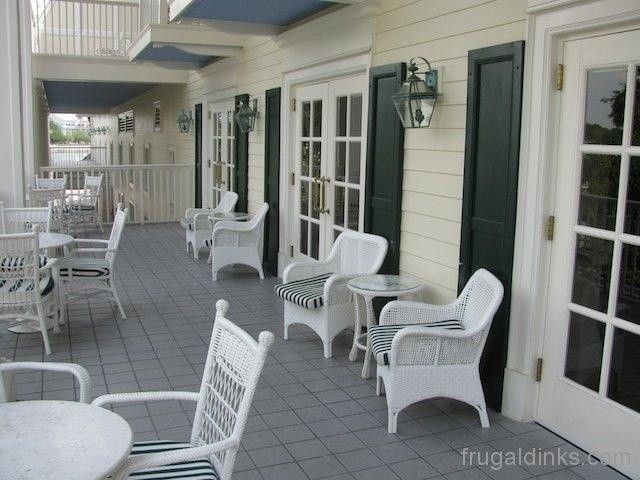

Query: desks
<box><xmin>0</xmin><ymin>401</ymin><xmax>134</xmax><ymax>480</ymax></box>
<box><xmin>208</xmin><ymin>211</ymin><xmax>250</xmax><ymax>266</ymax></box>
<box><xmin>348</xmin><ymin>272</ymin><xmax>424</xmax><ymax>376</ymax></box>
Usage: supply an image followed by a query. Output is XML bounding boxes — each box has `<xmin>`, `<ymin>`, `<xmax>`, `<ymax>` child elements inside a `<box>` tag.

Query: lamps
<box><xmin>393</xmin><ymin>54</ymin><xmax>438</xmax><ymax>131</ymax></box>
<box><xmin>175</xmin><ymin>109</ymin><xmax>192</xmax><ymax>134</ymax></box>
<box><xmin>234</xmin><ymin>90</ymin><xmax>258</xmax><ymax>134</ymax></box>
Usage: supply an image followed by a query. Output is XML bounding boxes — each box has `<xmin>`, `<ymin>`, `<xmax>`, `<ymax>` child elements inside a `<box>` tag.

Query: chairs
<box><xmin>182</xmin><ymin>191</ymin><xmax>239</xmax><ymax>260</ymax></box>
<box><xmin>95</xmin><ymin>296</ymin><xmax>278</xmax><ymax>480</ymax></box>
<box><xmin>207</xmin><ymin>201</ymin><xmax>270</xmax><ymax>283</ymax></box>
<box><xmin>2</xmin><ymin>172</ymin><xmax>136</xmax><ymax>357</ymax></box>
<box><xmin>1</xmin><ymin>358</ymin><xmax>93</xmax><ymax>409</ymax></box>
<box><xmin>368</xmin><ymin>268</ymin><xmax>508</xmax><ymax>436</ymax></box>
<box><xmin>272</xmin><ymin>231</ymin><xmax>388</xmax><ymax>361</ymax></box>
